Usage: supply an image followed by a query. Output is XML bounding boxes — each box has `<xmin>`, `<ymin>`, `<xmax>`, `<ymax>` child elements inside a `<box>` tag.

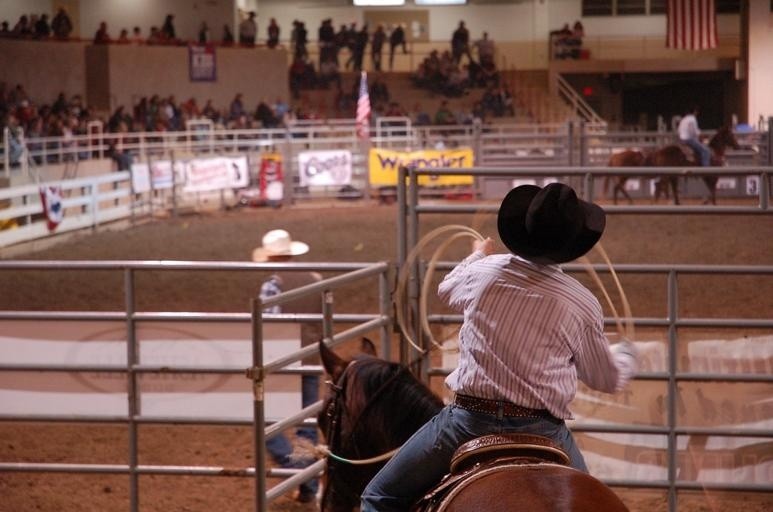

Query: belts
<box><xmin>453</xmin><ymin>395</ymin><xmax>548</xmax><ymax>420</ymax></box>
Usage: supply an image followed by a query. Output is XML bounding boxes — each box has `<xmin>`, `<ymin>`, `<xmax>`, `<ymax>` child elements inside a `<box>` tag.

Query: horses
<box><xmin>319</xmin><ymin>336</ymin><xmax>632</xmax><ymax>511</ymax></box>
<box><xmin>603</xmin><ymin>148</ymin><xmax>671</xmax><ymax>205</ymax></box>
<box><xmin>648</xmin><ymin>124</ymin><xmax>740</xmax><ymax>205</ymax></box>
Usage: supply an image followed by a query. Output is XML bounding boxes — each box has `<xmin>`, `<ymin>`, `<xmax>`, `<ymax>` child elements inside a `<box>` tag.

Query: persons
<box><xmin>359</xmin><ymin>182</ymin><xmax>636</xmax><ymax>512</ymax></box>
<box><xmin>555</xmin><ymin>21</ymin><xmax>584</xmax><ymax>59</ymax></box>
<box><xmin>676</xmin><ymin>106</ymin><xmax>711</xmax><ymax>171</ymax></box>
<box><xmin>0</xmin><ymin>8</ymin><xmax>280</xmax><ymax>52</ymax></box>
<box><xmin>288</xmin><ymin>15</ymin><xmax>514</xmax><ymax>137</ymax></box>
<box><xmin>0</xmin><ymin>83</ymin><xmax>317</xmax><ymax>170</ymax></box>
<box><xmin>248</xmin><ymin>228</ymin><xmax>331</xmax><ymax>501</ymax></box>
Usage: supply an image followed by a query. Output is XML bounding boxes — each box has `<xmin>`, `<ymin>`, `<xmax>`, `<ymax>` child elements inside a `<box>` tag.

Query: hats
<box><xmin>495</xmin><ymin>182</ymin><xmax>607</xmax><ymax>265</ymax></box>
<box><xmin>250</xmin><ymin>228</ymin><xmax>310</xmax><ymax>262</ymax></box>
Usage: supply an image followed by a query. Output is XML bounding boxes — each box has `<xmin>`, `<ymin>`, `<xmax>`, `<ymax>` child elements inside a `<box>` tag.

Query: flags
<box><xmin>666</xmin><ymin>0</ymin><xmax>719</xmax><ymax>50</ymax></box>
